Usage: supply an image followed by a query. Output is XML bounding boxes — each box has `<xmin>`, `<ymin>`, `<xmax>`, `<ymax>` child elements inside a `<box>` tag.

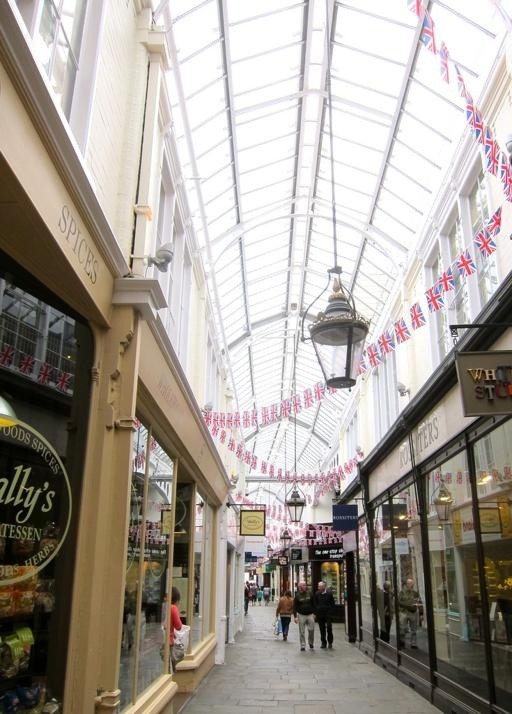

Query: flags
<box><xmin>125</xmin><ymin>416</ymin><xmax>202</xmax><ymax>544</ymax></box>
<box><xmin>18</xmin><ymin>352</ymin><xmax>37</xmax><ymax>376</ymax></box>
<box><xmin>409</xmin><ymin>0</ymin><xmax>512</xmax><ymax>202</ymax></box>
<box><xmin>235</xmin><ymin>494</ymin><xmax>342</xmax><ymax>546</ymax></box>
<box><xmin>356</xmin><ymin>205</ymin><xmax>501</xmax><ymax>373</ymax></box>
<box><xmin>55</xmin><ymin>369</ymin><xmax>74</xmax><ymax>394</ymax></box>
<box><xmin>36</xmin><ymin>362</ymin><xmax>55</xmax><ymax>386</ymax></box>
<box><xmin>212</xmin><ymin>426</ymin><xmax>364</xmax><ymax>504</ymax></box>
<box><xmin>205</xmin><ymin>380</ymin><xmax>337</xmax><ymax>429</ymax></box>
<box><xmin>0</xmin><ymin>341</ymin><xmax>17</xmax><ymax>368</ymax></box>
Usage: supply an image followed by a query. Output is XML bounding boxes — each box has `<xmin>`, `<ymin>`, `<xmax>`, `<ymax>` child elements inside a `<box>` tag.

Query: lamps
<box><xmin>431</xmin><ymin>466</ymin><xmax>454</xmax><ymax>521</ymax></box>
<box><xmin>299</xmin><ymin>2</ymin><xmax>368</xmax><ymax>389</ymax></box>
<box><xmin>267</xmin><ymin>333</ymin><xmax>306</xmax><ymax>558</ymax></box>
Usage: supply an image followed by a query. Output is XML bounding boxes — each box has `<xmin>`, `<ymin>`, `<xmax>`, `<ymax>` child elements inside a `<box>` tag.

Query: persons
<box><xmin>293</xmin><ymin>581</ymin><xmax>315</xmax><ymax>652</ymax></box>
<box><xmin>244</xmin><ymin>580</ymin><xmax>271</xmax><ymax>616</ymax></box>
<box><xmin>399</xmin><ymin>578</ymin><xmax>424</xmax><ymax>650</ymax></box>
<box><xmin>276</xmin><ymin>589</ymin><xmax>293</xmax><ymax>641</ymax></box>
<box><xmin>168</xmin><ymin>585</ymin><xmax>183</xmax><ymax>674</ymax></box>
<box><xmin>377</xmin><ymin>581</ymin><xmax>395</xmax><ymax>647</ymax></box>
<box><xmin>313</xmin><ymin>581</ymin><xmax>337</xmax><ymax>648</ymax></box>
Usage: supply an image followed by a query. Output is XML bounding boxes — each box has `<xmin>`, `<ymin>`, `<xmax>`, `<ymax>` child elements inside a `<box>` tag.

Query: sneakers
<box><xmin>283</xmin><ymin>634</ymin><xmax>288</xmax><ymax>640</ymax></box>
<box><xmin>321</xmin><ymin>643</ymin><xmax>332</xmax><ymax>648</ymax></box>
<box><xmin>411</xmin><ymin>645</ymin><xmax>418</xmax><ymax>649</ymax></box>
<box><xmin>301</xmin><ymin>644</ymin><xmax>313</xmax><ymax>651</ymax></box>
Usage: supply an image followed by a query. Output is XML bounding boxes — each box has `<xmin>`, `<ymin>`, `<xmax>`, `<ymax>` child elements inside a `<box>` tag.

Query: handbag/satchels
<box><xmin>272</xmin><ymin>619</ymin><xmax>282</xmax><ymax>634</ymax></box>
<box><xmin>172</xmin><ymin>643</ymin><xmax>185</xmax><ymax>664</ymax></box>
